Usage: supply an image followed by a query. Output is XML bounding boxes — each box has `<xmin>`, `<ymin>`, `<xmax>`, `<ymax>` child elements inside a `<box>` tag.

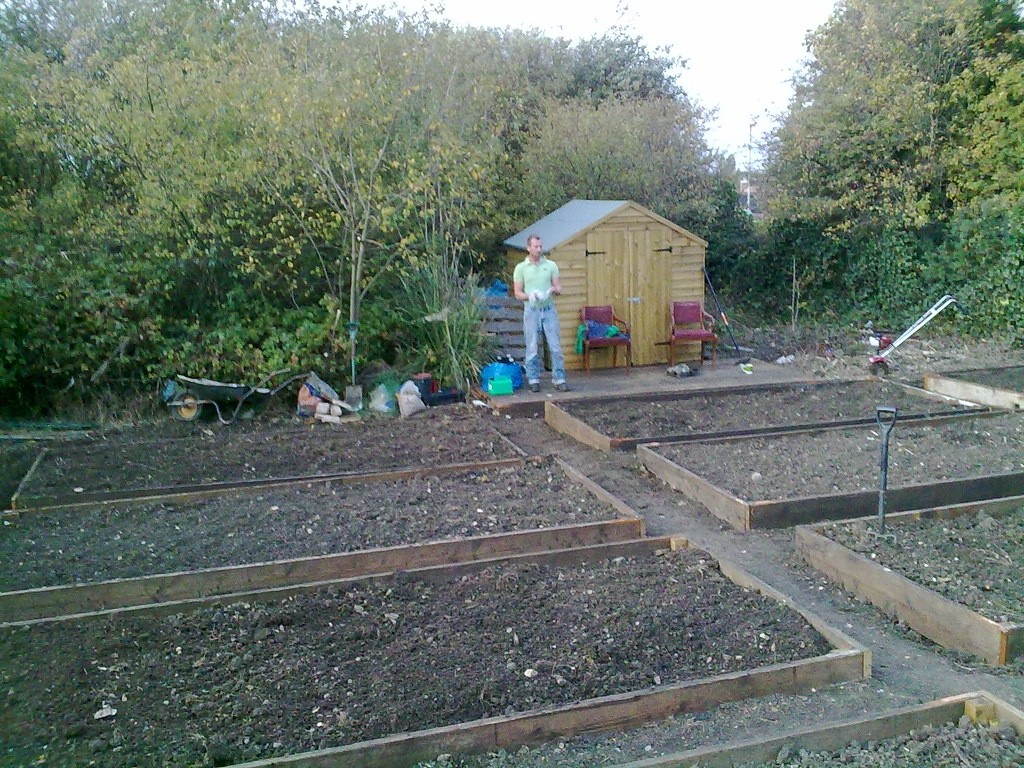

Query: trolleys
<box><xmin>166</xmin><ymin>367</ymin><xmax>312</xmax><ymax>425</ymax></box>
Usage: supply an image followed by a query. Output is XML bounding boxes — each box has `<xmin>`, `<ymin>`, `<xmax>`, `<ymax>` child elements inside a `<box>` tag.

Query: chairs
<box><xmin>581</xmin><ymin>304</ymin><xmax>631</xmax><ymax>372</ymax></box>
<box><xmin>671</xmin><ymin>300</ymin><xmax>718</xmax><ymax>369</ymax></box>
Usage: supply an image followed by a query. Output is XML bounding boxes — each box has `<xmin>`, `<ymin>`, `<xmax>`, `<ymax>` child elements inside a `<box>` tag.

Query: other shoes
<box><xmin>555</xmin><ymin>383</ymin><xmax>568</xmax><ymax>392</ymax></box>
<box><xmin>530</xmin><ymin>383</ymin><xmax>540</xmax><ymax>392</ymax></box>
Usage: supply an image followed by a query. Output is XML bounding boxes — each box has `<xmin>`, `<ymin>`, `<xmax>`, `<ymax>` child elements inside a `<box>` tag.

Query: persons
<box><xmin>513</xmin><ymin>235</ymin><xmax>570</xmax><ymax>392</ymax></box>
